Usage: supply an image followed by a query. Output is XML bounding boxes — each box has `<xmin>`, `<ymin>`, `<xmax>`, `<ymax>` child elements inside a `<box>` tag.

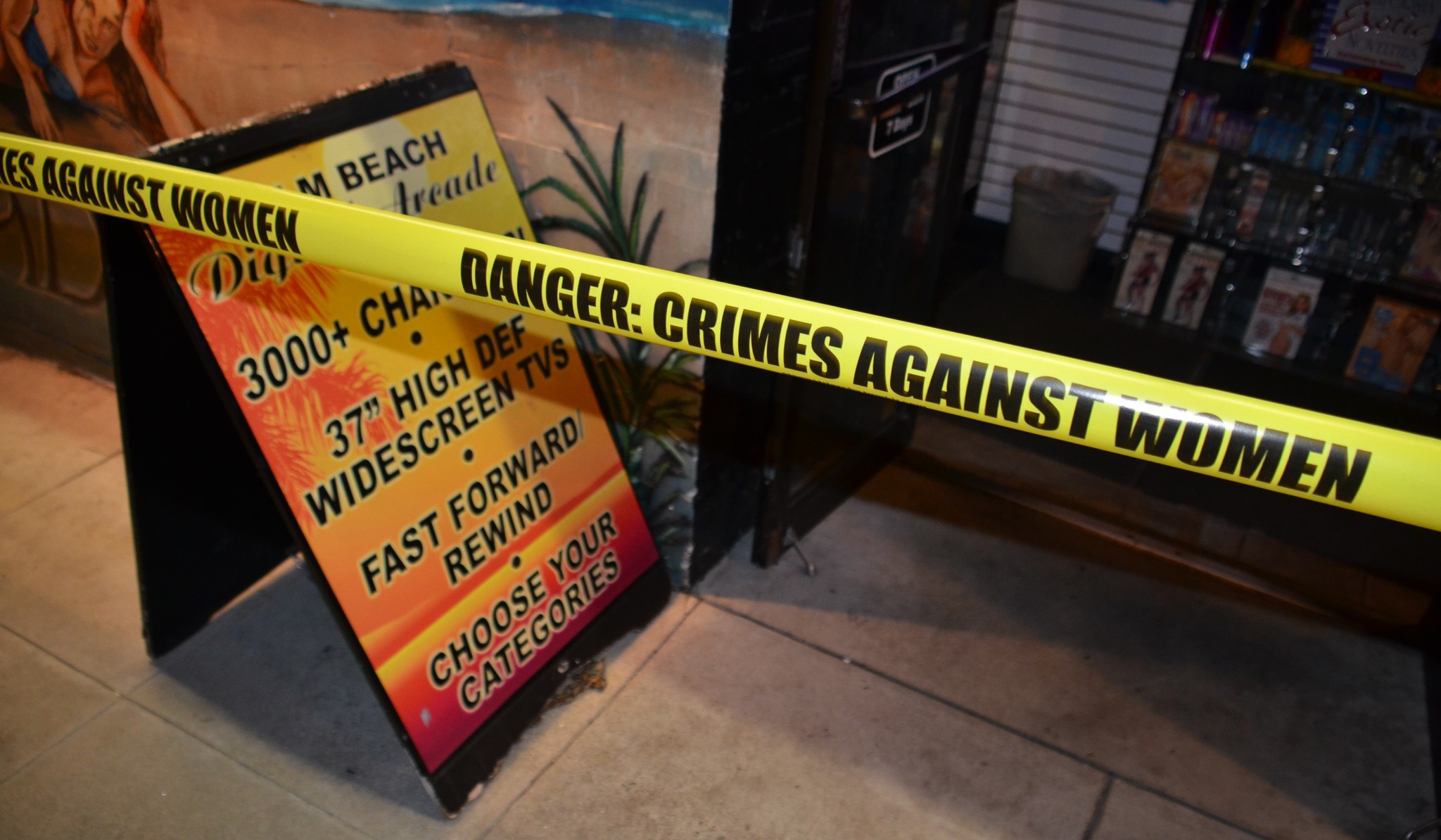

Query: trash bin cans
<box><xmin>1001</xmin><ymin>166</ymin><xmax>1121</xmax><ymax>291</ymax></box>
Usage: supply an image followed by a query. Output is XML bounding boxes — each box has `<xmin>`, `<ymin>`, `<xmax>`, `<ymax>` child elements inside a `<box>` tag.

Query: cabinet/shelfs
<box><xmin>1110</xmin><ymin>0</ymin><xmax>1441</xmax><ymax>397</ymax></box>
